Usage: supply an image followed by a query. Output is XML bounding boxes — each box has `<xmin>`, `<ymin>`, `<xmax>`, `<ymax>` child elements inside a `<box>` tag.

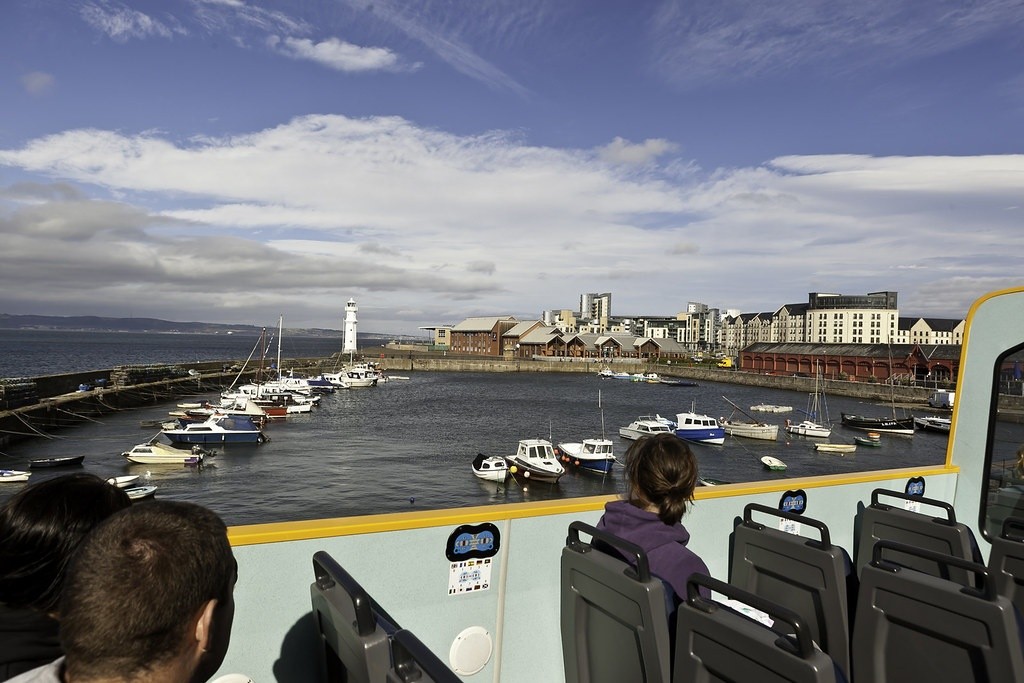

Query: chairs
<box><xmin>309</xmin><ymin>475</ymin><xmax>1024</xmax><ymax>683</ymax></box>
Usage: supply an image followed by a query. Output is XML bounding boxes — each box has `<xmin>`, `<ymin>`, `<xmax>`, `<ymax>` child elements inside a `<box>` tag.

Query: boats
<box><xmin>761</xmin><ymin>456</ymin><xmax>788</xmax><ymax>471</ymax></box>
<box><xmin>103</xmin><ymin>475</ymin><xmax>139</xmax><ymax>487</ymax></box>
<box><xmin>698</xmin><ymin>476</ymin><xmax>732</xmax><ymax>487</ymax></box>
<box><xmin>0</xmin><ymin>469</ymin><xmax>32</xmax><ymax>483</ymax></box>
<box><xmin>618</xmin><ymin>415</ymin><xmax>677</xmax><ymax>442</ymax></box>
<box><xmin>610</xmin><ymin>371</ymin><xmax>630</xmax><ymax>380</ymax></box>
<box><xmin>812</xmin><ymin>443</ymin><xmax>858</xmax><ymax>453</ymax></box>
<box><xmin>645</xmin><ymin>373</ymin><xmax>661</xmax><ymax>384</ymax></box>
<box><xmin>168</xmin><ymin>313</ymin><xmax>389</xmax><ymax>423</ymax></box>
<box><xmin>597</xmin><ymin>363</ymin><xmax>613</xmax><ymax>377</ymax></box>
<box><xmin>854</xmin><ymin>432</ymin><xmax>882</xmax><ymax>447</ymax></box>
<box><xmin>471</xmin><ymin>453</ymin><xmax>507</xmax><ymax>483</ymax></box>
<box><xmin>629</xmin><ymin>373</ymin><xmax>648</xmax><ymax>382</ymax></box>
<box><xmin>122</xmin><ymin>485</ymin><xmax>158</xmax><ymax>500</ymax></box>
<box><xmin>504</xmin><ymin>439</ymin><xmax>567</xmax><ymax>484</ymax></box>
<box><xmin>659</xmin><ymin>376</ymin><xmax>698</xmax><ymax>386</ymax></box>
<box><xmin>654</xmin><ymin>410</ymin><xmax>727</xmax><ymax>445</ymax></box>
<box><xmin>750</xmin><ymin>404</ymin><xmax>793</xmax><ymax>413</ymax></box>
<box><xmin>160</xmin><ymin>413</ymin><xmax>271</xmax><ymax>445</ymax></box>
<box><xmin>913</xmin><ymin>415</ymin><xmax>952</xmax><ymax>434</ymax></box>
<box><xmin>27</xmin><ymin>454</ymin><xmax>85</xmax><ymax>467</ymax></box>
<box><xmin>717</xmin><ymin>416</ymin><xmax>779</xmax><ymax>441</ymax></box>
<box><xmin>557</xmin><ymin>437</ymin><xmax>617</xmax><ymax>473</ymax></box>
<box><xmin>120</xmin><ymin>431</ymin><xmax>205</xmax><ymax>464</ymax></box>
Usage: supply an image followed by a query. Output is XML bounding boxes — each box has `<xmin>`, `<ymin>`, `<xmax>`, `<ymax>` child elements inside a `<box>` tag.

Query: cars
<box><xmin>691</xmin><ymin>351</ymin><xmax>726</xmax><ymax>362</ymax></box>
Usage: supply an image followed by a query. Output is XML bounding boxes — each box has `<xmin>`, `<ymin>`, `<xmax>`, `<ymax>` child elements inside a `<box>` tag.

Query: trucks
<box><xmin>717</xmin><ymin>357</ymin><xmax>736</xmax><ymax>369</ymax></box>
<box><xmin>927</xmin><ymin>389</ymin><xmax>956</xmax><ymax>409</ymax></box>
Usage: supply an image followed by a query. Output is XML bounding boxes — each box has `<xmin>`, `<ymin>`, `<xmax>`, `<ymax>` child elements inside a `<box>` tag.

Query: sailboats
<box><xmin>785</xmin><ymin>358</ymin><xmax>832</xmax><ymax>438</ymax></box>
<box><xmin>840</xmin><ymin>334</ymin><xmax>915</xmax><ymax>435</ymax></box>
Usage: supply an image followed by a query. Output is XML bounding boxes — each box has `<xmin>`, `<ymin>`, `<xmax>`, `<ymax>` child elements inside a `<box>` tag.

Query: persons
<box><xmin>591</xmin><ymin>432</ymin><xmax>711</xmax><ymax>601</ymax></box>
<box><xmin>0</xmin><ymin>472</ymin><xmax>132</xmax><ymax>683</ymax></box>
<box><xmin>5</xmin><ymin>501</ymin><xmax>239</xmax><ymax>683</ymax></box>
